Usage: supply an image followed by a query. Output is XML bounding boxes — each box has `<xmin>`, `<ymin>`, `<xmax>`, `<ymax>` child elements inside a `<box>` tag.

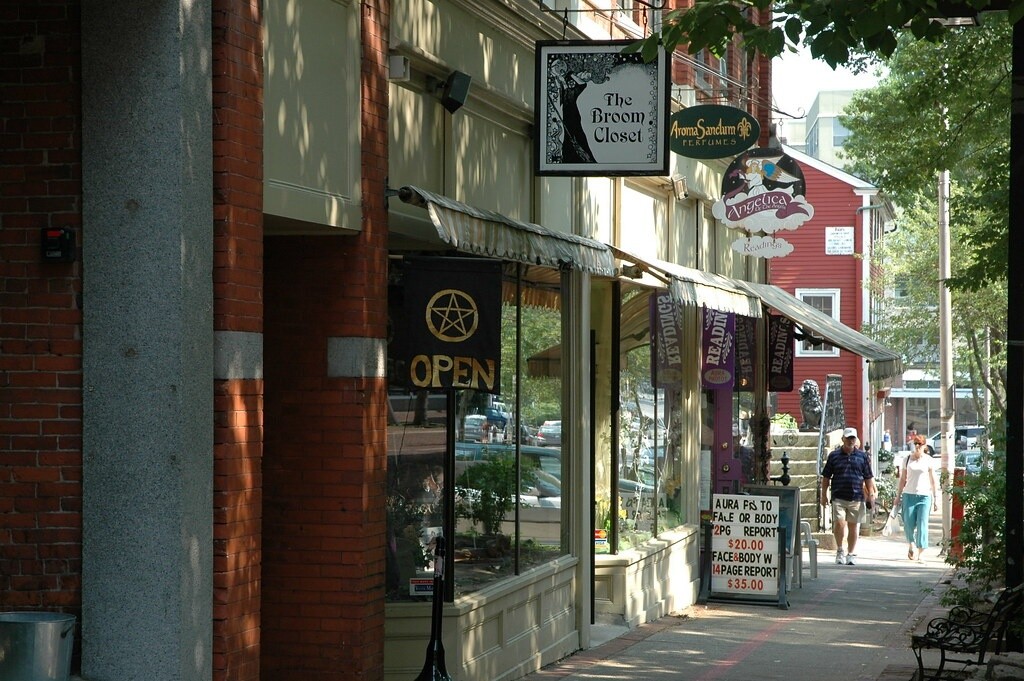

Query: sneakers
<box><xmin>836</xmin><ymin>548</ymin><xmax>844</xmax><ymax>564</ymax></box>
<box><xmin>846</xmin><ymin>553</ymin><xmax>856</xmax><ymax>565</ymax></box>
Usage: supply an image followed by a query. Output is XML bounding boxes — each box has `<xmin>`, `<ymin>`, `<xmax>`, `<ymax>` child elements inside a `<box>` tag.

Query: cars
<box><xmin>924</xmin><ymin>425</ymin><xmax>986</xmax><ymax>457</ymax></box>
<box><xmin>387</xmin><ymin>387</ymin><xmax>666</xmax><ymax>548</ymax></box>
<box><xmin>954</xmin><ymin>450</ymin><xmax>994</xmax><ymax>476</ymax></box>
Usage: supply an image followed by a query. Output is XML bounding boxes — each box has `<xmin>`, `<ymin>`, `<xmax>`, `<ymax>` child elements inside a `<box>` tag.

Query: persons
<box><xmin>894</xmin><ymin>434</ymin><xmax>938</xmax><ymax>564</ymax></box>
<box><xmin>906</xmin><ymin>430</ymin><xmax>916</xmax><ymax>452</ymax></box>
<box><xmin>482</xmin><ymin>422</ymin><xmax>543</xmax><ymax>447</ymax></box>
<box><xmin>821</xmin><ymin>428</ymin><xmax>877</xmax><ymax>565</ymax></box>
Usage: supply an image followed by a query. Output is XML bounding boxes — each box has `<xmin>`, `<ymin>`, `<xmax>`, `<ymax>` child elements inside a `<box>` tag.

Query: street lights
<box><xmin>918</xmin><ymin>12</ymin><xmax>982</xmax><ymax>560</ymax></box>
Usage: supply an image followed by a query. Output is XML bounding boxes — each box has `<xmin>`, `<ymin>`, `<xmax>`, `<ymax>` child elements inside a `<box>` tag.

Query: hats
<box><xmin>844</xmin><ymin>428</ymin><xmax>857</xmax><ymax>438</ymax></box>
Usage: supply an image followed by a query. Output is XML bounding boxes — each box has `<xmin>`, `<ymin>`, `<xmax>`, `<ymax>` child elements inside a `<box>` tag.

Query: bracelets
<box><xmin>868</xmin><ymin>493</ymin><xmax>873</xmax><ymax>496</ymax></box>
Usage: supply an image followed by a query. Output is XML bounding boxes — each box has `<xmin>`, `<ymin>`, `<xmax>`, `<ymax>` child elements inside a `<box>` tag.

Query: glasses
<box><xmin>913</xmin><ymin>442</ymin><xmax>924</xmax><ymax>446</ymax></box>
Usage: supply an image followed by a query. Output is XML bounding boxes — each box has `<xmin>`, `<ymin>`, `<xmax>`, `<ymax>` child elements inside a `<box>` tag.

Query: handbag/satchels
<box><xmin>881</xmin><ymin>504</ymin><xmax>901</xmax><ymax>538</ymax></box>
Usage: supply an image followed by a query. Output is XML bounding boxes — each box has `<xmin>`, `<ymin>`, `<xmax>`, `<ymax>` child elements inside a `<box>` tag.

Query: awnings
<box><xmin>399</xmin><ymin>186</ymin><xmax>620</xmax><ymax>277</ymax></box>
<box><xmin>718</xmin><ymin>274</ymin><xmax>905</xmax><ymax>381</ymax></box>
<box><xmin>501</xmin><ymin>261</ymin><xmax>562</xmax><ymax>311</ymax></box>
<box><xmin>527</xmin><ymin>289</ymin><xmax>657</xmax><ymax>380</ymax></box>
<box><xmin>603</xmin><ymin>243</ymin><xmax>762</xmax><ymax>318</ymax></box>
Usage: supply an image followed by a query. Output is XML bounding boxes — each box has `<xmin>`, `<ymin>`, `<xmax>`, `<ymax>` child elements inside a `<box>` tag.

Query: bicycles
<box><xmin>867</xmin><ymin>499</ymin><xmax>889</xmax><ymax>532</ymax></box>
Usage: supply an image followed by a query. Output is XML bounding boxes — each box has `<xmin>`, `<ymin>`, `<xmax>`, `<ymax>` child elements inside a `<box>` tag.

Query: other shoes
<box><xmin>908</xmin><ymin>547</ymin><xmax>914</xmax><ymax>560</ymax></box>
<box><xmin>918</xmin><ymin>556</ymin><xmax>923</xmax><ymax>564</ymax></box>
